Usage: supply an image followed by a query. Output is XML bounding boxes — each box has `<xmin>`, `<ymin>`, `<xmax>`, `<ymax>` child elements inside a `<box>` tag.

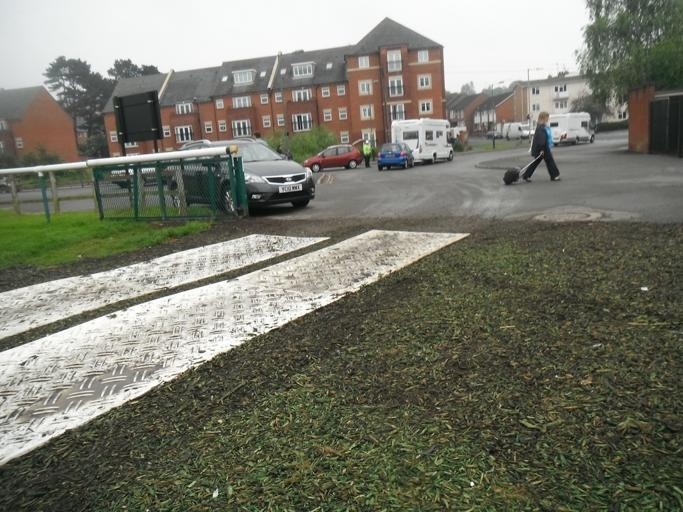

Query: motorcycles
<box><xmin>276</xmin><ymin>143</ymin><xmax>295</xmax><ymax>161</ymax></box>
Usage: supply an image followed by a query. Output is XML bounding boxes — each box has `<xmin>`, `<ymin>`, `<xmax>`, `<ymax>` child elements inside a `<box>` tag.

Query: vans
<box><xmin>390</xmin><ymin>117</ymin><xmax>454</xmax><ymax>165</ymax></box>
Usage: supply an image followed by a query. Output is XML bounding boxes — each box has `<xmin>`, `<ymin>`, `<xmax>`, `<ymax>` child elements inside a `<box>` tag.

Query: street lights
<box><xmin>490</xmin><ymin>78</ymin><xmax>505</xmax><ymax>149</ymax></box>
<box><xmin>526</xmin><ymin>66</ymin><xmax>541</xmax><ymax>141</ymax></box>
<box><xmin>315</xmin><ymin>82</ymin><xmax>323</xmax><ymax>126</ymax></box>
<box><xmin>371</xmin><ymin>78</ymin><xmax>389</xmax><ymax>143</ymax></box>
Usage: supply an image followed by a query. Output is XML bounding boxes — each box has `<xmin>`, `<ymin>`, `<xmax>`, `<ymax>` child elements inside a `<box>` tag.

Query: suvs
<box><xmin>165</xmin><ymin>135</ymin><xmax>317</xmax><ymax>218</ymax></box>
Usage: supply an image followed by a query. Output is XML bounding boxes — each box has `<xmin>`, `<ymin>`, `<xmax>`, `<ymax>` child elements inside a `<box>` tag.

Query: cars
<box><xmin>374</xmin><ymin>142</ymin><xmax>415</xmax><ymax>172</ymax></box>
<box><xmin>450</xmin><ymin>110</ymin><xmax>596</xmax><ymax>151</ymax></box>
<box><xmin>300</xmin><ymin>138</ymin><xmax>368</xmax><ymax>173</ymax></box>
<box><xmin>107</xmin><ymin>163</ymin><xmax>157</xmax><ymax>189</ymax></box>
<box><xmin>0</xmin><ymin>174</ymin><xmax>21</xmax><ymax>194</ymax></box>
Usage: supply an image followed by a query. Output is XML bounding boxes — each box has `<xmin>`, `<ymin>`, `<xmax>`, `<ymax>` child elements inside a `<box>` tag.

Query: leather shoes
<box><xmin>524</xmin><ymin>177</ymin><xmax>531</xmax><ymax>182</ymax></box>
<box><xmin>550</xmin><ymin>176</ymin><xmax>560</xmax><ymax>181</ymax></box>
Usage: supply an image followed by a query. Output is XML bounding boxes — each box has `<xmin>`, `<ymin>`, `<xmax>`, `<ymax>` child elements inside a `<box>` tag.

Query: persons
<box><xmin>522</xmin><ymin>112</ymin><xmax>561</xmax><ymax>182</ymax></box>
<box><xmin>276</xmin><ymin>145</ymin><xmax>284</xmax><ymax>158</ymax></box>
<box><xmin>253</xmin><ymin>131</ymin><xmax>268</xmax><ymax>146</ymax></box>
<box><xmin>362</xmin><ymin>140</ymin><xmax>372</xmax><ymax>168</ymax></box>
<box><xmin>285</xmin><ymin>150</ymin><xmax>293</xmax><ymax>160</ymax></box>
